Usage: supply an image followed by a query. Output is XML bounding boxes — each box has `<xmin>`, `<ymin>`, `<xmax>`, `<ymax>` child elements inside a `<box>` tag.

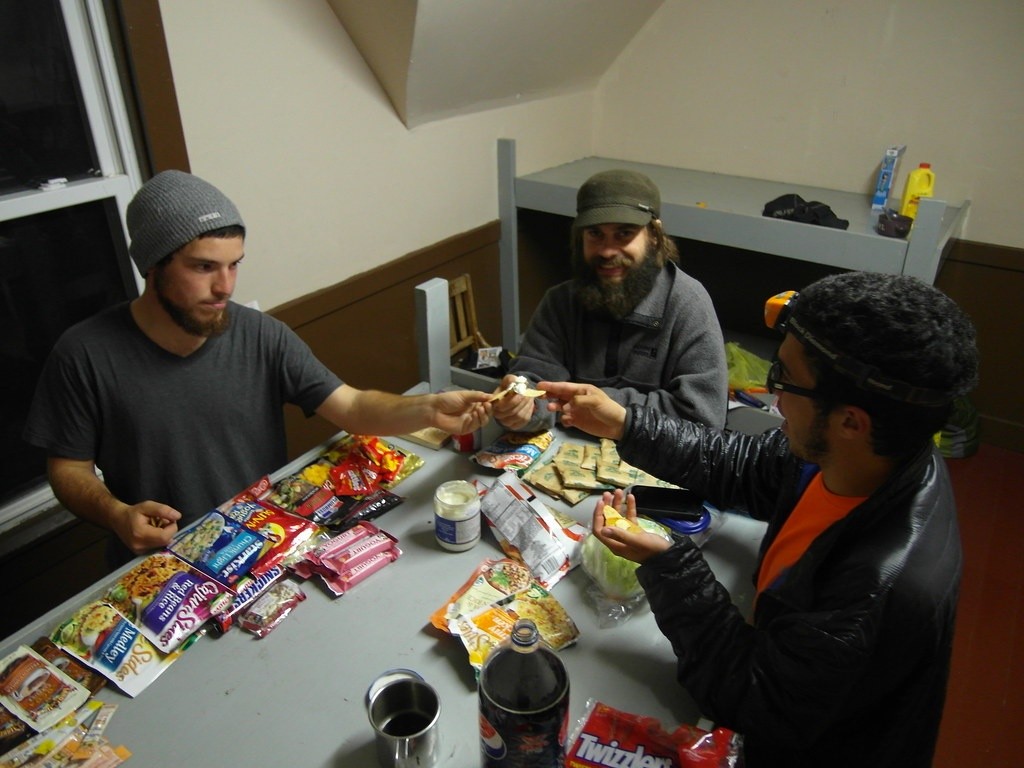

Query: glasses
<box><xmin>766</xmin><ymin>365</ymin><xmax>867</xmax><ymax>413</ymax></box>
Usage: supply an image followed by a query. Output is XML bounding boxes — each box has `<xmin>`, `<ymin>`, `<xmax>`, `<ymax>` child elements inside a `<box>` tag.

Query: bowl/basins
<box><xmin>623</xmin><ymin>480</ymin><xmax>727</xmax><ymax>549</ymax></box>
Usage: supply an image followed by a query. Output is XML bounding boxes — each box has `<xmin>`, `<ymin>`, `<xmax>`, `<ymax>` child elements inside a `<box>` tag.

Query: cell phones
<box><xmin>630</xmin><ymin>485</ymin><xmax>703</xmax><ymax>521</ymax></box>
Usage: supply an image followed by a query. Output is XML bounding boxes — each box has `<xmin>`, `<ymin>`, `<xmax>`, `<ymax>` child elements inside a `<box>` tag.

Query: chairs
<box><xmin>450</xmin><ymin>271</ymin><xmax>515</xmax><ymax>380</ymax></box>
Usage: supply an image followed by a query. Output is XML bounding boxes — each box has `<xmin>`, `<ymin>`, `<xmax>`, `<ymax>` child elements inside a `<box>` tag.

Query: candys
<box><xmin>291</xmin><ymin>520</ymin><xmax>403</xmax><ymax>596</ymax></box>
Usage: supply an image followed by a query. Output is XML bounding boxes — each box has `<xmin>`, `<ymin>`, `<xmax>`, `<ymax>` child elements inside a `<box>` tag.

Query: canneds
<box><xmin>432</xmin><ymin>480</ymin><xmax>482</xmax><ymax>552</ymax></box>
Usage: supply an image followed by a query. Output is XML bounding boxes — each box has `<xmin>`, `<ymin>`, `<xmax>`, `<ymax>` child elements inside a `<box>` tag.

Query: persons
<box><xmin>488</xmin><ymin>169</ymin><xmax>729</xmax><ymax>433</ymax></box>
<box><xmin>536</xmin><ymin>270</ymin><xmax>977</xmax><ymax>768</ymax></box>
<box><xmin>26</xmin><ymin>170</ymin><xmax>495</xmax><ymax>568</ymax></box>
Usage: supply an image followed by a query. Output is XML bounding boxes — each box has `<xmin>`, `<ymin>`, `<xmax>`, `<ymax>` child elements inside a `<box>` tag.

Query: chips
<box><xmin>603</xmin><ymin>504</ymin><xmax>645</xmax><ymax>535</ymax></box>
<box><xmin>513</xmin><ymin>384</ymin><xmax>548</xmax><ymax>398</ymax></box>
<box><xmin>487</xmin><ymin>387</ymin><xmax>511</xmax><ymax>402</ymax></box>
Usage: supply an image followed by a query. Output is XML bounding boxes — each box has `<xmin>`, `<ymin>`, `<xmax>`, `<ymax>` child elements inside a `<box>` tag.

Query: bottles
<box><xmin>477</xmin><ymin>618</ymin><xmax>570</xmax><ymax>768</ymax></box>
<box><xmin>434</xmin><ymin>480</ymin><xmax>482</xmax><ymax>552</ymax></box>
<box><xmin>899</xmin><ymin>163</ymin><xmax>935</xmax><ymax>219</ymax></box>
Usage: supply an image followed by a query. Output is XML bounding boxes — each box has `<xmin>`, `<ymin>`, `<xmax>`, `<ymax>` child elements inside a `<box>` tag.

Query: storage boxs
<box><xmin>877</xmin><ymin>215</ymin><xmax>914</xmax><ymax>238</ymax></box>
<box><xmin>871</xmin><ymin>144</ymin><xmax>908</xmax><ymax>211</ymax></box>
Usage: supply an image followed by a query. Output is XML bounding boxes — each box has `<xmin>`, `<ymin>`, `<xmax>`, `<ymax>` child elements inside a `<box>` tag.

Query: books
<box><xmin>397</xmin><ymin>427</ymin><xmax>453</xmax><ymax>451</ymax></box>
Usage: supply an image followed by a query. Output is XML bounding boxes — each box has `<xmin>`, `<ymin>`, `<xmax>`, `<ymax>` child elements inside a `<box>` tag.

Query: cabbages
<box><xmin>579</xmin><ymin>518</ymin><xmax>673</xmax><ymax>600</ymax></box>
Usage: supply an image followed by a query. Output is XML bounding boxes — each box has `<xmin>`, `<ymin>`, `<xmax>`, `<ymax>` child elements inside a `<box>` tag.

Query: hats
<box><xmin>575</xmin><ymin>168</ymin><xmax>660</xmax><ymax>230</ymax></box>
<box><xmin>126</xmin><ymin>170</ymin><xmax>245</xmax><ymax>277</ymax></box>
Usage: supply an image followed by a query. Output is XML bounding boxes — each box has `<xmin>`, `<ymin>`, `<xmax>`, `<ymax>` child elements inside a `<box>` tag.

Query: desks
<box><xmin>0</xmin><ymin>375</ymin><xmax>773</xmax><ymax>768</ymax></box>
<box><xmin>499</xmin><ymin>152</ymin><xmax>973</xmax><ymax>376</ymax></box>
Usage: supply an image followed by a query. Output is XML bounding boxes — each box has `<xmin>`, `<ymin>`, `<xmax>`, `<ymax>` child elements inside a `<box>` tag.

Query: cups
<box><xmin>369</xmin><ymin>678</ymin><xmax>442</xmax><ymax>768</ymax></box>
